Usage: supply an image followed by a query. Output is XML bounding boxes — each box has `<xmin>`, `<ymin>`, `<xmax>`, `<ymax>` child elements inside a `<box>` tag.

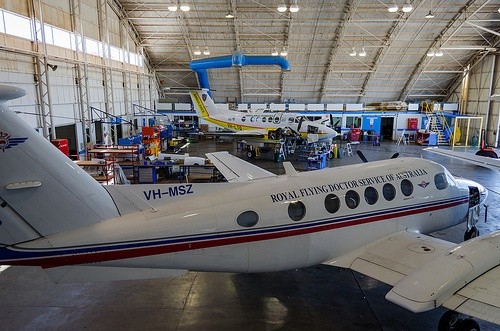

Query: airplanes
<box><xmin>0</xmin><ymin>83</ymin><xmax>500</xmax><ymax>331</ymax></box>
<box><xmin>188</xmin><ymin>90</ymin><xmax>338</xmax><ymax>158</ymax></box>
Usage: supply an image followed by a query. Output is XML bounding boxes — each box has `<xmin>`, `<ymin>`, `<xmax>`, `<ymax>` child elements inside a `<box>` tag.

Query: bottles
<box><xmin>144</xmin><ymin>160</ymin><xmax>147</xmax><ymax>166</ymax></box>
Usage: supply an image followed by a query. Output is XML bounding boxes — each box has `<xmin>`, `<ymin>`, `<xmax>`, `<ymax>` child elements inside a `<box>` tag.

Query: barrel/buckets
<box><xmin>371</xmin><ymin>130</ymin><xmax>374</xmax><ymax>135</ymax></box>
<box><xmin>363</xmin><ymin>135</ymin><xmax>367</xmax><ymax>141</ymax></box>
<box><xmin>343</xmin><ymin>133</ymin><xmax>348</xmax><ymax>139</ymax></box>
<box><xmin>274</xmin><ymin>152</ymin><xmax>281</xmax><ymax>163</ymax></box>
<box><xmin>370</xmin><ymin>136</ymin><xmax>374</xmax><ymax>141</ymax></box>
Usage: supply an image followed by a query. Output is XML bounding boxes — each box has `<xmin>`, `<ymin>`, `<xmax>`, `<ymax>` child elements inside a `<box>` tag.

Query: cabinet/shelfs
<box><xmin>74</xmin><ymin>144</ymin><xmax>140</xmax><ymax>185</ymax></box>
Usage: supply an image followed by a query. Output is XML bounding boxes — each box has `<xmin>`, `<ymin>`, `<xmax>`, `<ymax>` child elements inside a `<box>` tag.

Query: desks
<box><xmin>135</xmin><ymin>163</ymin><xmax>221</xmax><ymax>182</ymax></box>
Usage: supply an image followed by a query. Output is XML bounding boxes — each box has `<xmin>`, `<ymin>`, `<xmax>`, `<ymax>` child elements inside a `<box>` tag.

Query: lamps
<box><xmin>425</xmin><ymin>0</ymin><xmax>435</xmax><ymax>18</ymax></box>
<box><xmin>167</xmin><ymin>1</ymin><xmax>178</xmax><ymax>11</ymax></box>
<box><xmin>180</xmin><ymin>0</ymin><xmax>191</xmax><ymax>12</ymax></box>
<box><xmin>402</xmin><ymin>0</ymin><xmax>412</xmax><ymax>12</ymax></box>
<box><xmin>47</xmin><ymin>62</ymin><xmax>58</xmax><ymax>71</ymax></box>
<box><xmin>271</xmin><ymin>40</ymin><xmax>278</xmax><ymax>55</ymax></box>
<box><xmin>290</xmin><ymin>0</ymin><xmax>299</xmax><ymax>12</ymax></box>
<box><xmin>277</xmin><ymin>0</ymin><xmax>287</xmax><ymax>12</ymax></box>
<box><xmin>388</xmin><ymin>0</ymin><xmax>399</xmax><ymax>12</ymax></box>
<box><xmin>193</xmin><ymin>32</ymin><xmax>201</xmax><ymax>55</ymax></box>
<box><xmin>428</xmin><ymin>33</ymin><xmax>444</xmax><ymax>57</ymax></box>
<box><xmin>203</xmin><ymin>32</ymin><xmax>210</xmax><ymax>55</ymax></box>
<box><xmin>280</xmin><ymin>39</ymin><xmax>288</xmax><ymax>56</ymax></box>
<box><xmin>358</xmin><ymin>33</ymin><xmax>367</xmax><ymax>56</ymax></box>
<box><xmin>224</xmin><ymin>8</ymin><xmax>235</xmax><ymax>19</ymax></box>
<box><xmin>349</xmin><ymin>35</ymin><xmax>357</xmax><ymax>56</ymax></box>
